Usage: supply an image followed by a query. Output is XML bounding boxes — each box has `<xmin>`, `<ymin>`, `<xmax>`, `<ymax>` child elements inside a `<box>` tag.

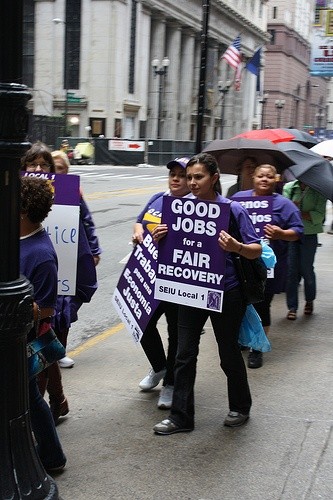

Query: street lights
<box><xmin>258</xmin><ymin>93</ymin><xmax>269</xmax><ymax>130</ymax></box>
<box><xmin>274</xmin><ymin>99</ymin><xmax>286</xmax><ymax>128</ymax></box>
<box><xmin>315</xmin><ymin>113</ymin><xmax>324</xmax><ymax>137</ymax></box>
<box><xmin>217</xmin><ymin>80</ymin><xmax>233</xmax><ymax>140</ymax></box>
<box><xmin>151</xmin><ymin>56</ymin><xmax>170</xmax><ymax>138</ymax></box>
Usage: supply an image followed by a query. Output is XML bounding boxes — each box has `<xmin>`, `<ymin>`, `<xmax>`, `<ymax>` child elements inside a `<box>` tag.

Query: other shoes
<box><xmin>37</xmin><ymin>447</ymin><xmax>66</xmax><ymax>473</ymax></box>
<box><xmin>59</xmin><ymin>356</ymin><xmax>75</xmax><ymax>367</ymax></box>
<box><xmin>287</xmin><ymin>310</ymin><xmax>297</xmax><ymax>320</ymax></box>
<box><xmin>247</xmin><ymin>349</ymin><xmax>263</xmax><ymax>368</ymax></box>
<box><xmin>157</xmin><ymin>385</ymin><xmax>174</xmax><ymax>409</ymax></box>
<box><xmin>138</xmin><ymin>368</ymin><xmax>167</xmax><ymax>390</ymax></box>
<box><xmin>153</xmin><ymin>418</ymin><xmax>194</xmax><ymax>435</ymax></box>
<box><xmin>224</xmin><ymin>410</ymin><xmax>249</xmax><ymax>426</ymax></box>
<box><xmin>305</xmin><ymin>301</ymin><xmax>313</xmax><ymax>314</ymax></box>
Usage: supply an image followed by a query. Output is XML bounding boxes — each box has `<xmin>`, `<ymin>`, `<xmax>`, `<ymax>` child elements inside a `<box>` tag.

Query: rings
<box><xmin>154</xmin><ymin>234</ymin><xmax>159</xmax><ymax>238</ymax></box>
<box><xmin>222</xmin><ymin>237</ymin><xmax>225</xmax><ymax>239</ymax></box>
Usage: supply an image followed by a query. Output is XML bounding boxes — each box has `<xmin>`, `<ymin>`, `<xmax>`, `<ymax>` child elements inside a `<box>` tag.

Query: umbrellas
<box><xmin>232</xmin><ymin>129</ymin><xmax>296</xmax><ymax>143</ymax></box>
<box><xmin>283</xmin><ymin>127</ymin><xmax>319</xmax><ymax>144</ymax></box>
<box><xmin>309</xmin><ymin>138</ymin><xmax>333</xmax><ymax>158</ymax></box>
<box><xmin>277</xmin><ymin>141</ymin><xmax>333</xmax><ymax>202</ymax></box>
<box><xmin>202</xmin><ymin>139</ymin><xmax>296</xmax><ymax>174</ymax></box>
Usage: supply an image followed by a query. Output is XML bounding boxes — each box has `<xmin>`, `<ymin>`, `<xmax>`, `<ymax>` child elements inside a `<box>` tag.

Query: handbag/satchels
<box><xmin>231</xmin><ymin>250</ymin><xmax>268</xmax><ymax>304</ymax></box>
<box><xmin>24</xmin><ymin>325</ymin><xmax>66</xmax><ymax>380</ymax></box>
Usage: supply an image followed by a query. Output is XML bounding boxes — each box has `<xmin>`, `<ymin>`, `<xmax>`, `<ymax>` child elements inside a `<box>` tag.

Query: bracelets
<box><xmin>239</xmin><ymin>243</ymin><xmax>243</xmax><ymax>253</ymax></box>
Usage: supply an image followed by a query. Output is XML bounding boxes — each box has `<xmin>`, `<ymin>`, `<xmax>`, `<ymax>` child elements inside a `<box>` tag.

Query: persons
<box><xmin>153</xmin><ymin>154</ymin><xmax>264</xmax><ymax>435</ymax></box>
<box><xmin>285</xmin><ymin>180</ymin><xmax>328</xmax><ymax>319</ymax></box>
<box><xmin>51</xmin><ymin>149</ymin><xmax>101</xmax><ymax>265</ymax></box>
<box><xmin>229</xmin><ymin>163</ymin><xmax>303</xmax><ymax>367</ymax></box>
<box><xmin>21</xmin><ymin>145</ymin><xmax>97</xmax><ymax>367</ymax></box>
<box><xmin>21</xmin><ymin>176</ymin><xmax>70</xmax><ymax>426</ymax></box>
<box><xmin>26</xmin><ymin>331</ymin><xmax>67</xmax><ymax>474</ymax></box>
<box><xmin>223</xmin><ymin>158</ymin><xmax>257</xmax><ymax>197</ymax></box>
<box><xmin>132</xmin><ymin>157</ymin><xmax>198</xmax><ymax>409</ymax></box>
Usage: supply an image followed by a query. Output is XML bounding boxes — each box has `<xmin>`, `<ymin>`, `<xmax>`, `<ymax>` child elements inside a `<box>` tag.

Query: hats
<box><xmin>166</xmin><ymin>157</ymin><xmax>189</xmax><ymax>168</ymax></box>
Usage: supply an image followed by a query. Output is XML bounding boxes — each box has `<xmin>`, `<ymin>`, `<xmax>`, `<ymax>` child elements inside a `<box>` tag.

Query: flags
<box><xmin>223</xmin><ymin>34</ymin><xmax>243</xmax><ymax>92</ymax></box>
<box><xmin>246</xmin><ymin>48</ymin><xmax>261</xmax><ymax>77</ymax></box>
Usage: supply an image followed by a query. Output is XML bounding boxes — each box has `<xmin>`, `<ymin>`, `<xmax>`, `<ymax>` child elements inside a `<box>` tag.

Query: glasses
<box><xmin>169</xmin><ymin>172</ymin><xmax>186</xmax><ymax>179</ymax></box>
<box><xmin>24</xmin><ymin>160</ymin><xmax>51</xmax><ymax>169</ymax></box>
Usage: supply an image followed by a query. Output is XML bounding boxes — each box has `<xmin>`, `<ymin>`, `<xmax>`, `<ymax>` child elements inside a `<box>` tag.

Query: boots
<box><xmin>39</xmin><ymin>371</ymin><xmax>48</xmax><ymax>397</ymax></box>
<box><xmin>47</xmin><ymin>361</ymin><xmax>70</xmax><ymax>419</ymax></box>
